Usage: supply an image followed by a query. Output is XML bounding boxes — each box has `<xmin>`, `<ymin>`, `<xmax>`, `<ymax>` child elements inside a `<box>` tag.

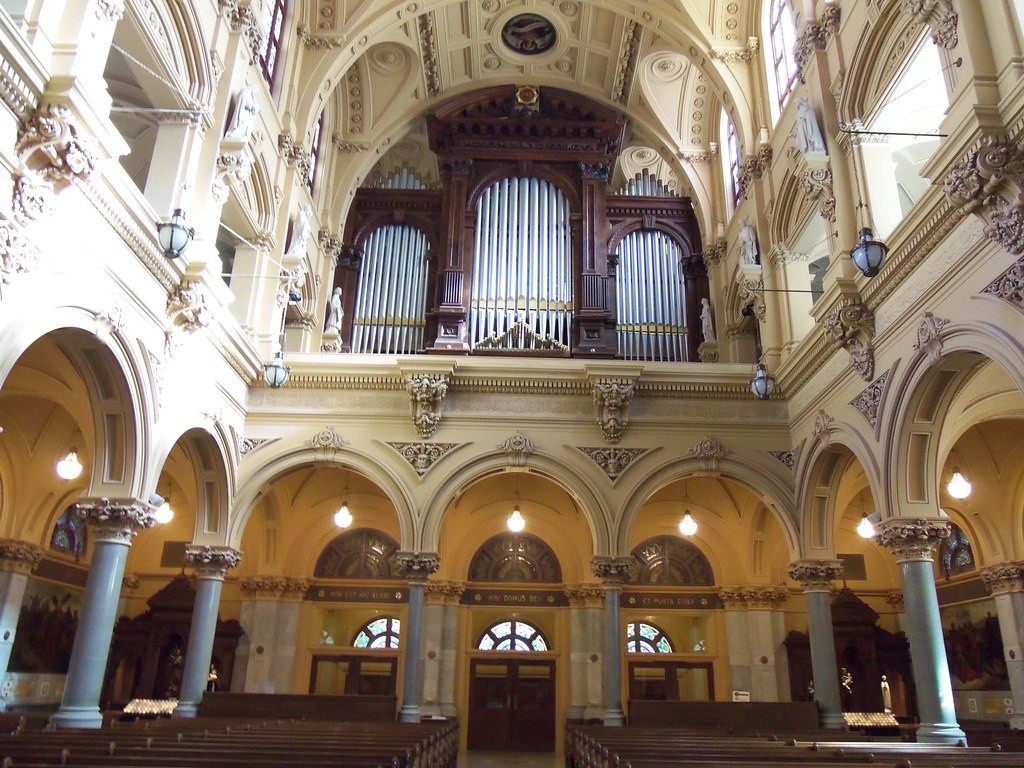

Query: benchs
<box><xmin>565</xmin><ymin>699</ymin><xmax>1024</xmax><ymax>768</ymax></box>
<box><xmin>0</xmin><ymin>691</ymin><xmax>460</xmax><ymax>768</ymax></box>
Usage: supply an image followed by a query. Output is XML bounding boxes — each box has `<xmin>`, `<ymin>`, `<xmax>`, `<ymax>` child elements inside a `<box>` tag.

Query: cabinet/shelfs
<box><xmin>99</xmin><ymin>569</ymin><xmax>244</xmax><ymax>711</ymax></box>
<box><xmin>783</xmin><ymin>577</ymin><xmax>921</xmax><ymax>722</ymax></box>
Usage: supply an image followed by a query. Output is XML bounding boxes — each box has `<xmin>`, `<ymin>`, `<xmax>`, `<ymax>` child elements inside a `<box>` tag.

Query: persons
<box><xmin>224</xmin><ymin>82</ymin><xmax>260</xmax><ymax>139</ymax></box>
<box><xmin>324</xmin><ymin>287</ymin><xmax>344</xmax><ymax>333</ymax></box>
<box><xmin>736</xmin><ymin>217</ymin><xmax>758</xmax><ymax>266</ymax></box>
<box><xmin>285</xmin><ymin>203</ymin><xmax>313</xmax><ymax>257</ymax></box>
<box><xmin>700</xmin><ymin>298</ymin><xmax>716</xmax><ymax>343</ymax></box>
<box><xmin>788</xmin><ymin>95</ymin><xmax>827</xmax><ymax>157</ymax></box>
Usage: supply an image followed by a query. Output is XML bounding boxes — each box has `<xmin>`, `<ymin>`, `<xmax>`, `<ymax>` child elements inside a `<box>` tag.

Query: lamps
<box><xmin>155</xmin><ymin>476</ymin><xmax>175</xmax><ymax>525</ymax></box>
<box><xmin>836</xmin><ymin>120</ymin><xmax>949</xmax><ymax>277</ymax></box>
<box><xmin>856</xmin><ymin>489</ymin><xmax>876</xmax><ymax>540</ymax></box>
<box><xmin>222</xmin><ymin>270</ymin><xmax>301</xmax><ymax>389</ymax></box>
<box><xmin>505</xmin><ymin>474</ymin><xmax>525</xmax><ymax>534</ymax></box>
<box><xmin>679</xmin><ymin>479</ymin><xmax>698</xmax><ymax>537</ymax></box>
<box><xmin>56</xmin><ymin>427</ymin><xmax>84</xmax><ymax>481</ymax></box>
<box><xmin>946</xmin><ymin>448</ymin><xmax>972</xmax><ymax>499</ymax></box>
<box><xmin>111</xmin><ymin>102</ymin><xmax>216</xmax><ymax>259</ymax></box>
<box><xmin>333</xmin><ymin>471</ymin><xmax>353</xmax><ymax>529</ymax></box>
<box><xmin>746</xmin><ymin>281</ymin><xmax>825</xmax><ymax>400</ymax></box>
